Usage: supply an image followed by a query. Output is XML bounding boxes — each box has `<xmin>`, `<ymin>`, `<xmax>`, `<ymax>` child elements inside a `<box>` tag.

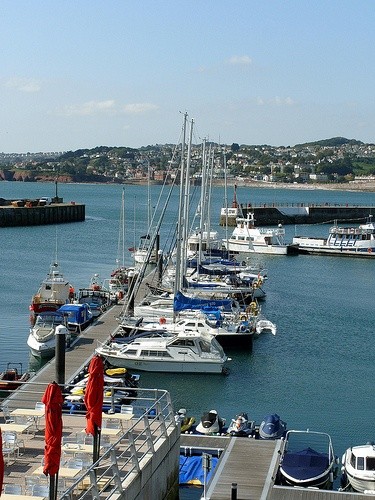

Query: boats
<box><xmin>222</xmin><ymin>212</ymin><xmax>300</xmax><ymax>255</ymax></box>
<box><xmin>226</xmin><ymin>411</ymin><xmax>256</xmax><ymax>437</ymax></box>
<box><xmin>278</xmin><ymin>429</ymin><xmax>340</xmax><ymax>489</ymax></box>
<box><xmin>174</xmin><ymin>408</ymin><xmax>195</xmax><ymax>432</ymax></box>
<box><xmin>195</xmin><ymin>409</ymin><xmax>227</xmax><ymax>434</ymax></box>
<box><xmin>258</xmin><ymin>414</ymin><xmax>288</xmax><ymax>439</ymax></box>
<box><xmin>0</xmin><ymin>362</ymin><xmax>31</xmax><ymax>395</ymax></box>
<box><xmin>292</xmin><ymin>225</ymin><xmax>375</xmax><ymax>259</ymax></box>
<box><xmin>342</xmin><ymin>443</ymin><xmax>375</xmax><ymax>493</ymax></box>
<box><xmin>63</xmin><ymin>367</ymin><xmax>141</xmax><ymax>410</ymax></box>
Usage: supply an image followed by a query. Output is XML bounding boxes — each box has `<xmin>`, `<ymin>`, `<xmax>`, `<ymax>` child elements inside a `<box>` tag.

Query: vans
<box><xmin>39</xmin><ymin>197</ymin><xmax>52</xmax><ymax>205</ymax></box>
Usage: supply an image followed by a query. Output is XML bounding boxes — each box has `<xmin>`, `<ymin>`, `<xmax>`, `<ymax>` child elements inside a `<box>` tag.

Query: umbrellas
<box><xmin>84</xmin><ymin>354</ymin><xmax>103</xmax><ymax>468</ymax></box>
<box><xmin>41</xmin><ymin>381</ymin><xmax>63</xmax><ymax>500</ymax></box>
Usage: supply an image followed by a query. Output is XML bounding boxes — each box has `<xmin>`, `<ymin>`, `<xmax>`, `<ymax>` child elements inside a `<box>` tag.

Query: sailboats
<box><xmin>25</xmin><ymin>111</ymin><xmax>269</xmax><ymax>375</ymax></box>
